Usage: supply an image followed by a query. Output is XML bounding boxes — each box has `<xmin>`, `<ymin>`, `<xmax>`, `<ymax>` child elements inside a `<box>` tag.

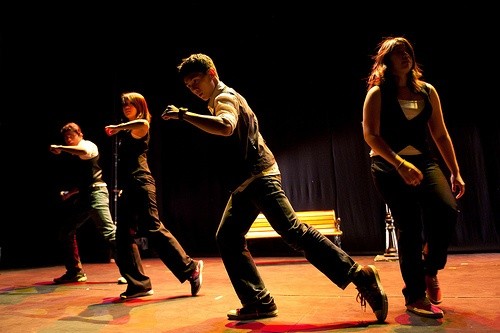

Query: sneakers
<box><xmin>227</xmin><ymin>297</ymin><xmax>278</xmax><ymax>319</ymax></box>
<box><xmin>355</xmin><ymin>264</ymin><xmax>387</xmax><ymax>323</ymax></box>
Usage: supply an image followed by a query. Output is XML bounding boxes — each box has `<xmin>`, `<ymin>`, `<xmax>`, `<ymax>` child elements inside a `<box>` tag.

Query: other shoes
<box><xmin>408</xmin><ymin>296</ymin><xmax>443</xmax><ymax>318</ymax></box>
<box><xmin>187</xmin><ymin>259</ymin><xmax>204</xmax><ymax>295</ymax></box>
<box><xmin>53</xmin><ymin>273</ymin><xmax>86</xmax><ymax>283</ymax></box>
<box><xmin>425</xmin><ymin>272</ymin><xmax>443</xmax><ymax>304</ymax></box>
<box><xmin>118</xmin><ymin>276</ymin><xmax>127</xmax><ymax>284</ymax></box>
<box><xmin>120</xmin><ymin>287</ymin><xmax>153</xmax><ymax>299</ymax></box>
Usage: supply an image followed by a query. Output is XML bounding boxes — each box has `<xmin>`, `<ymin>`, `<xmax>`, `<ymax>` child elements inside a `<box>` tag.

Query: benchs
<box><xmin>243</xmin><ymin>209</ymin><xmax>343</xmax><ymax>249</ymax></box>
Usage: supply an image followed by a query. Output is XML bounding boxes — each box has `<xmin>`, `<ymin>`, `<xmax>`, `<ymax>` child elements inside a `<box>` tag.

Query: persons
<box><xmin>363</xmin><ymin>38</ymin><xmax>465</xmax><ymax>318</ymax></box>
<box><xmin>49</xmin><ymin>122</ymin><xmax>128</xmax><ymax>284</ymax></box>
<box><xmin>104</xmin><ymin>92</ymin><xmax>202</xmax><ymax>297</ymax></box>
<box><xmin>161</xmin><ymin>53</ymin><xmax>388</xmax><ymax>322</ymax></box>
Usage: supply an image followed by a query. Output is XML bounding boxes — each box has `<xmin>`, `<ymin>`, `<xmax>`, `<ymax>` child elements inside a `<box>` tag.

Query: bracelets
<box><xmin>396</xmin><ymin>159</ymin><xmax>405</xmax><ymax>170</ymax></box>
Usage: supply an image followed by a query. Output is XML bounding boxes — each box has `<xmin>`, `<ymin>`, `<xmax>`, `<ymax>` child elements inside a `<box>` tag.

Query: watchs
<box><xmin>178</xmin><ymin>107</ymin><xmax>188</xmax><ymax>121</ymax></box>
